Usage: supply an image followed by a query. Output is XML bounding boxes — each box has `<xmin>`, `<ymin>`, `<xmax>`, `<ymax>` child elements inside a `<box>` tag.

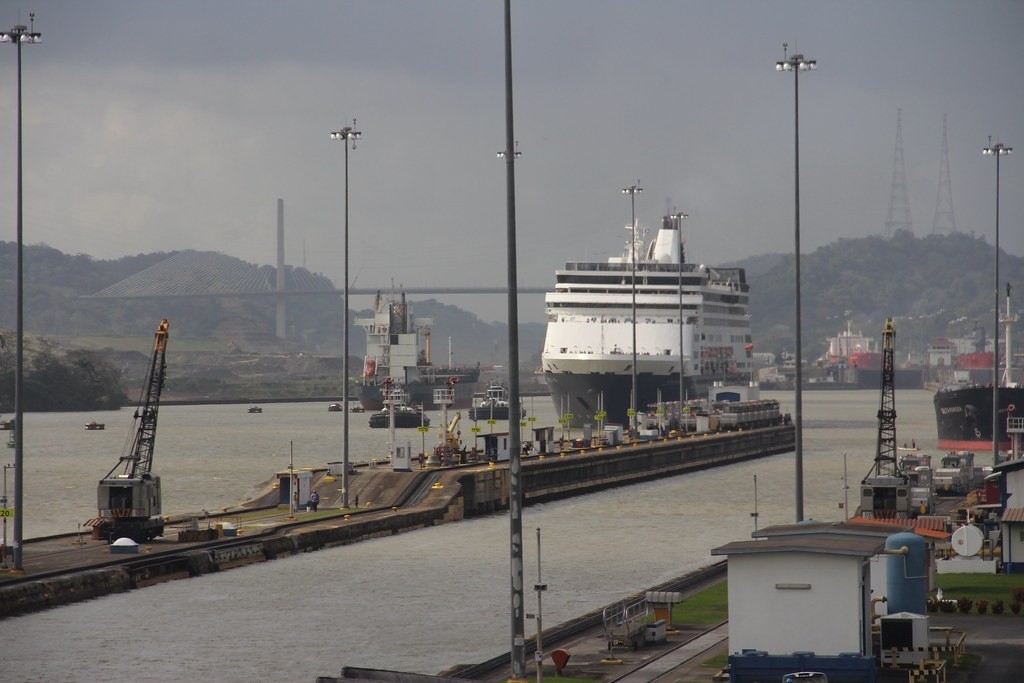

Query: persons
<box><xmin>311</xmin><ymin>489</ymin><xmax>319</xmax><ymax>512</ymax></box>
<box><xmin>293</xmin><ymin>491</ymin><xmax>299</xmax><ymax>512</ymax></box>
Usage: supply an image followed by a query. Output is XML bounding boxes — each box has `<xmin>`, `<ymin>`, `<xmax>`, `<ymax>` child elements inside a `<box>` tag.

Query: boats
<box><xmin>248</xmin><ymin>406</ymin><xmax>262</xmax><ymax>413</ymax></box>
<box><xmin>86</xmin><ymin>422</ymin><xmax>105</xmax><ymax>431</ymax></box>
<box><xmin>469</xmin><ymin>376</ymin><xmax>526</xmax><ymax>420</ymax></box>
<box><xmin>368</xmin><ymin>378</ymin><xmax>429</xmax><ymax>428</ymax></box>
<box><xmin>350</xmin><ymin>406</ymin><xmax>364</xmax><ymax>414</ymax></box>
<box><xmin>329</xmin><ymin>404</ymin><xmax>342</xmax><ymax>412</ymax></box>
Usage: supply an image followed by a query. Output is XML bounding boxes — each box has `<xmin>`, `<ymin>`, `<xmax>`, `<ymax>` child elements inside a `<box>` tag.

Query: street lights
<box><xmin>671</xmin><ymin>210</ymin><xmax>689</xmax><ymax>410</ymax></box>
<box><xmin>333</xmin><ymin>126</ymin><xmax>363</xmax><ymax>508</ymax></box>
<box><xmin>496</xmin><ymin>145</ymin><xmax>522</xmax><ymax>675</ymax></box>
<box><xmin>0</xmin><ymin>26</ymin><xmax>43</xmax><ymax>567</ymax></box>
<box><xmin>981</xmin><ymin>141</ymin><xmax>1013</xmax><ymax>470</ymax></box>
<box><xmin>622</xmin><ymin>184</ymin><xmax>643</xmax><ymax>429</ymax></box>
<box><xmin>777</xmin><ymin>54</ymin><xmax>816</xmax><ymax>523</ymax></box>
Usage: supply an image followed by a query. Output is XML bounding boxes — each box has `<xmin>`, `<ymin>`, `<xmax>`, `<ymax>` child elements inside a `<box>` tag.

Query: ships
<box><xmin>760</xmin><ymin>320</ymin><xmax>924</xmax><ymax>390</ymax></box>
<box><xmin>355</xmin><ymin>277</ymin><xmax>481</xmax><ymax>410</ymax></box>
<box><xmin>933</xmin><ymin>282</ymin><xmax>1024</xmax><ymax>451</ymax></box>
<box><xmin>539</xmin><ymin>213</ymin><xmax>755</xmax><ymax>429</ymax></box>
